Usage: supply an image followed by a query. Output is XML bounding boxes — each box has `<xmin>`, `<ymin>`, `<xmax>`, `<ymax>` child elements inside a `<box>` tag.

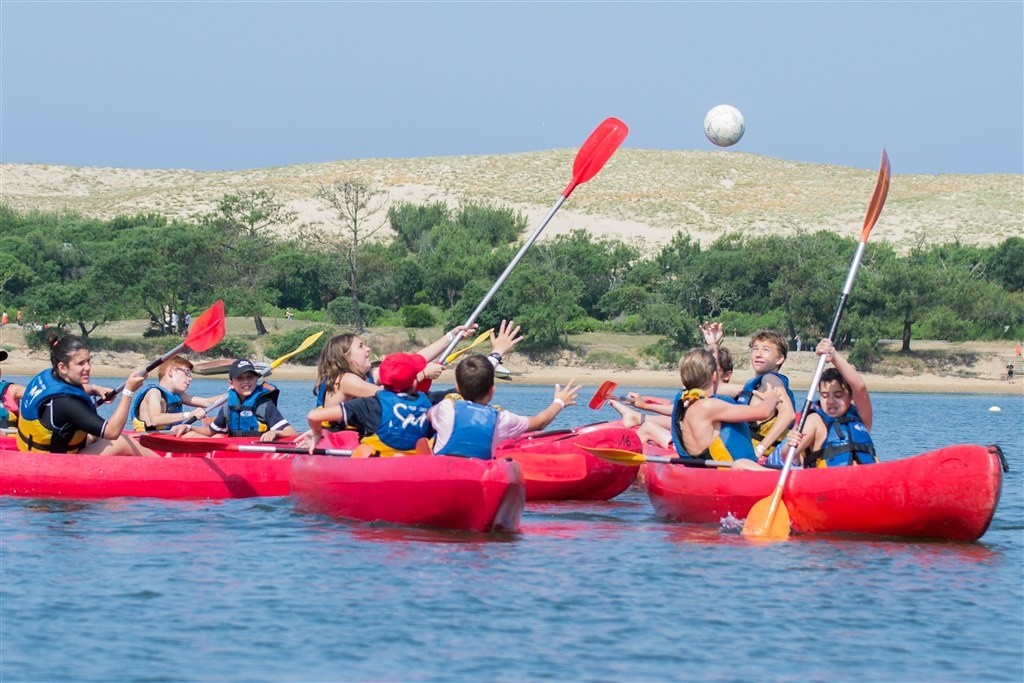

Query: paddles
<box><xmin>92</xmin><ymin>299</ymin><xmax>226</xmax><ymax>408</ymax></box>
<box><xmin>183</xmin><ymin>330</ymin><xmax>325</xmax><ymax>426</ymax></box>
<box><xmin>139</xmin><ymin>434</ymin><xmax>412</xmax><ymax>457</ymax></box>
<box><xmin>440</xmin><ymin>328</ymin><xmax>495</xmax><ymax>365</ymax></box>
<box><xmin>588</xmin><ymin>380</ymin><xmax>663</xmax><ymax>410</ymax></box>
<box><xmin>435</xmin><ymin>115</ymin><xmax>629</xmax><ymax>365</ymax></box>
<box><xmin>738</xmin><ymin>145</ymin><xmax>892</xmax><ymax>540</ymax></box>
<box><xmin>574</xmin><ymin>443</ymin><xmax>805</xmax><ymax>471</ymax></box>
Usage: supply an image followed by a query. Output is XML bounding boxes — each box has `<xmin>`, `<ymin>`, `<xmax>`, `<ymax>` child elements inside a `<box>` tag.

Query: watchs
<box><xmin>488</xmin><ymin>353</ymin><xmax>504</xmax><ymax>365</ymax></box>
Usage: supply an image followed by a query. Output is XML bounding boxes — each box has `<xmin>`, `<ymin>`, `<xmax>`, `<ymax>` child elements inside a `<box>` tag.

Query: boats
<box><xmin>323</xmin><ymin>410</ymin><xmax>644</xmax><ymax>503</ymax></box>
<box><xmin>0</xmin><ymin>408</ymin><xmax>322</xmax><ymax>501</ymax></box>
<box><xmin>289</xmin><ymin>426</ymin><xmax>528</xmax><ymax>535</ymax></box>
<box><xmin>638</xmin><ymin>435</ymin><xmax>1010</xmax><ymax>545</ymax></box>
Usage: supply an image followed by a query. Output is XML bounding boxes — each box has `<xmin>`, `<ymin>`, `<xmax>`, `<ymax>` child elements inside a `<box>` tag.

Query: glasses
<box><xmin>174</xmin><ymin>367</ymin><xmax>192</xmax><ymax>376</ymax></box>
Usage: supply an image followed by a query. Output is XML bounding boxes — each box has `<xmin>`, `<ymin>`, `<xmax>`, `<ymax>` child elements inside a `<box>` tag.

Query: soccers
<box><xmin>703</xmin><ymin>104</ymin><xmax>746</xmax><ymax>147</ymax></box>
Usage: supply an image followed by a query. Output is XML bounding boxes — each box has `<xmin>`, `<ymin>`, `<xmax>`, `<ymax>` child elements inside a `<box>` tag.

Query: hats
<box><xmin>379</xmin><ymin>352</ymin><xmax>428</xmax><ymax>393</ymax></box>
<box><xmin>229</xmin><ymin>359</ymin><xmax>259</xmax><ymax>379</ymax></box>
<box><xmin>0</xmin><ymin>350</ymin><xmax>8</xmax><ymax>361</ymax></box>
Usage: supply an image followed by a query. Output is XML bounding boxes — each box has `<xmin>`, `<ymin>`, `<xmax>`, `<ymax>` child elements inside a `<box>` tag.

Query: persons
<box><xmin>14</xmin><ymin>339</ymin><xmax>161</xmax><ymax>458</ymax></box>
<box><xmin>0</xmin><ymin>310</ymin><xmax>8</xmax><ymax>327</ymax></box>
<box><xmin>0</xmin><ymin>348</ymin><xmax>26</xmax><ymax>418</ymax></box>
<box><xmin>607</xmin><ymin>320</ymin><xmax>880</xmax><ymax>471</ymax></box>
<box><xmin>130</xmin><ymin>356</ymin><xmax>299</xmax><ymax>443</ymax></box>
<box><xmin>1006</xmin><ymin>361</ymin><xmax>1014</xmax><ymax>384</ymax></box>
<box><xmin>147</xmin><ymin>301</ymin><xmax>192</xmax><ymax>337</ymax></box>
<box><xmin>17</xmin><ymin>308</ymin><xmax>24</xmax><ymax>330</ymax></box>
<box><xmin>1014</xmin><ymin>342</ymin><xmax>1022</xmax><ymax>360</ymax></box>
<box><xmin>33</xmin><ymin>323</ymin><xmax>48</xmax><ymax>332</ymax></box>
<box><xmin>294</xmin><ymin>318</ymin><xmax>581</xmax><ymax>460</ymax></box>
<box><xmin>285</xmin><ymin>309</ymin><xmax>294</xmax><ymax>319</ymax></box>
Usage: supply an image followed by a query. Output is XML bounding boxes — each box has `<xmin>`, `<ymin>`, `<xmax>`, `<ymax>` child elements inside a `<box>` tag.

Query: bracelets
<box><xmin>416</xmin><ymin>378</ymin><xmax>420</xmax><ymax>384</ymax></box>
<box><xmin>422</xmin><ymin>369</ymin><xmax>426</xmax><ymax>380</ymax></box>
<box><xmin>123</xmin><ymin>387</ymin><xmax>138</xmax><ymax>400</ymax></box>
<box><xmin>183</xmin><ymin>412</ymin><xmax>190</xmax><ymax>420</ymax></box>
<box><xmin>446</xmin><ymin>330</ymin><xmax>454</xmax><ymax>343</ymax></box>
<box><xmin>487</xmin><ymin>356</ymin><xmax>500</xmax><ymax>368</ymax></box>
<box><xmin>186</xmin><ymin>423</ymin><xmax>192</xmax><ymax>432</ymax></box>
<box><xmin>552</xmin><ymin>398</ymin><xmax>566</xmax><ymax>408</ymax></box>
